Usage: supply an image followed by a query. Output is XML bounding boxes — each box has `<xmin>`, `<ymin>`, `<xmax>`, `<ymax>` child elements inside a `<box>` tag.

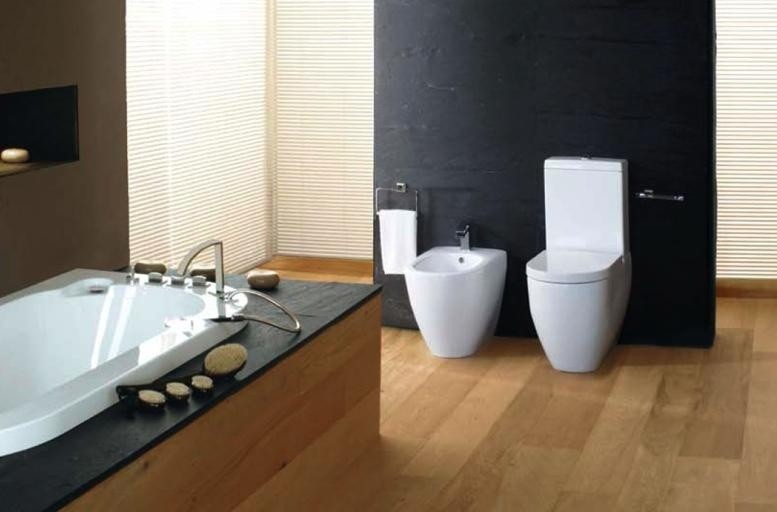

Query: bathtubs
<box><xmin>0</xmin><ymin>265</ymin><xmax>252</xmax><ymax>460</ymax></box>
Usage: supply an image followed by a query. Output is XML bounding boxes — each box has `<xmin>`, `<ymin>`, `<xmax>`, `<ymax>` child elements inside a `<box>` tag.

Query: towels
<box><xmin>378</xmin><ymin>208</ymin><xmax>418</xmax><ymax>275</ymax></box>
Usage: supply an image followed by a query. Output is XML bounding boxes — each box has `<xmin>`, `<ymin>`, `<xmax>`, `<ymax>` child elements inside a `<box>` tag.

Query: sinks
<box><xmin>399</xmin><ymin>242</ymin><xmax>510</xmax><ymax>360</ymax></box>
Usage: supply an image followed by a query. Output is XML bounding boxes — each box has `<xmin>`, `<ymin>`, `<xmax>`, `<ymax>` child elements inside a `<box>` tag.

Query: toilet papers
<box><xmin>374</xmin><ymin>200</ymin><xmax>422</xmax><ymax>278</ymax></box>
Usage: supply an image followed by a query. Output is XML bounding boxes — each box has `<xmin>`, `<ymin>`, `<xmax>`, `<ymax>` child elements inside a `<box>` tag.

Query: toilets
<box><xmin>522</xmin><ymin>149</ymin><xmax>637</xmax><ymax>376</ymax></box>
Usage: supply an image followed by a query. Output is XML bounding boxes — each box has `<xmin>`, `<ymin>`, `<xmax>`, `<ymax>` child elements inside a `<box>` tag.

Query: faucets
<box><xmin>453</xmin><ymin>217</ymin><xmax>475</xmax><ymax>254</ymax></box>
<box><xmin>177</xmin><ymin>238</ymin><xmax>225</xmax><ymax>302</ymax></box>
